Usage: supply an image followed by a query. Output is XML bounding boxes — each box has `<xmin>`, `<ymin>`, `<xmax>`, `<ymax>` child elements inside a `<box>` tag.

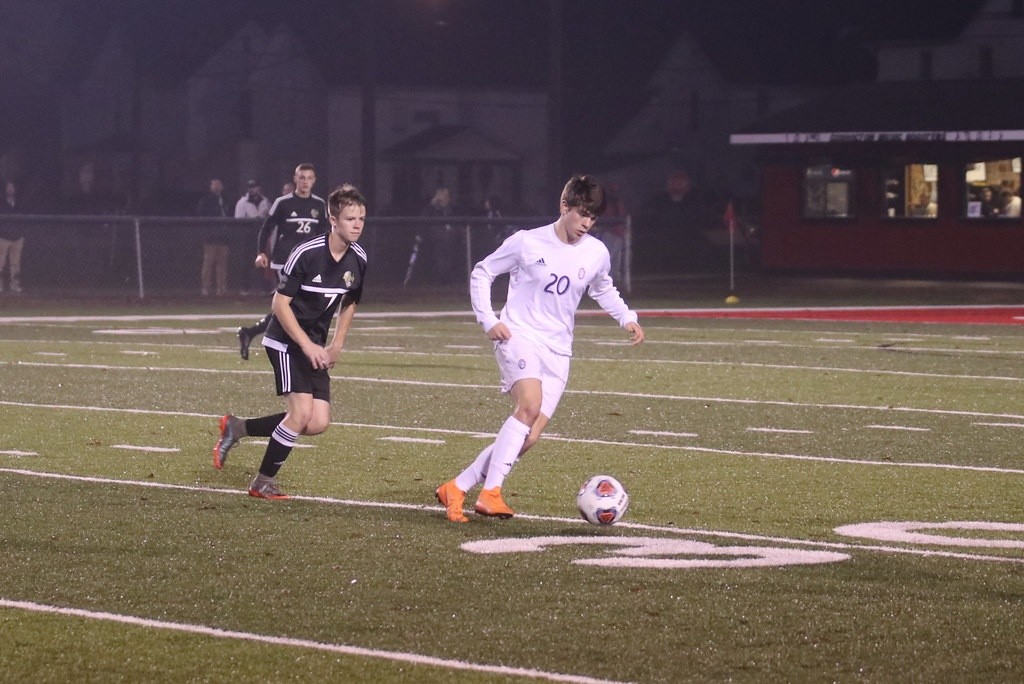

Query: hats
<box><xmin>247</xmin><ymin>177</ymin><xmax>259</xmax><ymax>188</ymax></box>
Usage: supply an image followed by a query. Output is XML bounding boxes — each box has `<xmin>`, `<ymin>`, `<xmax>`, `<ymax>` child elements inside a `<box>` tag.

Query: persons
<box><xmin>0</xmin><ymin>182</ymin><xmax>25</xmax><ymax>292</ymax></box>
<box><xmin>435</xmin><ymin>176</ymin><xmax>645</xmax><ymax>524</ymax></box>
<box><xmin>198</xmin><ymin>178</ymin><xmax>294</xmax><ymax>297</ymax></box>
<box><xmin>909</xmin><ymin>182</ymin><xmax>1022</xmax><ymax>218</ymax></box>
<box><xmin>236</xmin><ymin>162</ymin><xmax>329</xmax><ymax>359</ymax></box>
<box><xmin>423</xmin><ymin>188</ymin><xmax>516</xmax><ymax>299</ymax></box>
<box><xmin>213</xmin><ymin>183</ymin><xmax>369</xmax><ymax>501</ymax></box>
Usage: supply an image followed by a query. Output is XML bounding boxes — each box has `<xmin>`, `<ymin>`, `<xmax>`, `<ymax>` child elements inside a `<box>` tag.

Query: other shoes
<box><xmin>10</xmin><ymin>285</ymin><xmax>22</xmax><ymax>292</ymax></box>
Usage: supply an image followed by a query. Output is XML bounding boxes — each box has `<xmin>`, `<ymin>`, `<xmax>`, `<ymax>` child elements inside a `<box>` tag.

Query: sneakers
<box><xmin>213</xmin><ymin>415</ymin><xmax>248</xmax><ymax>470</ymax></box>
<box><xmin>435</xmin><ymin>478</ymin><xmax>469</xmax><ymax>522</ymax></box>
<box><xmin>249</xmin><ymin>472</ymin><xmax>291</xmax><ymax>499</ymax></box>
<box><xmin>237</xmin><ymin>326</ymin><xmax>252</xmax><ymax>360</ymax></box>
<box><xmin>474</xmin><ymin>486</ymin><xmax>513</xmax><ymax>519</ymax></box>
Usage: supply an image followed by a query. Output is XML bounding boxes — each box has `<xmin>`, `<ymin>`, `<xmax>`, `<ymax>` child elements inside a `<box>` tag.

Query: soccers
<box><xmin>575</xmin><ymin>474</ymin><xmax>630</xmax><ymax>525</ymax></box>
